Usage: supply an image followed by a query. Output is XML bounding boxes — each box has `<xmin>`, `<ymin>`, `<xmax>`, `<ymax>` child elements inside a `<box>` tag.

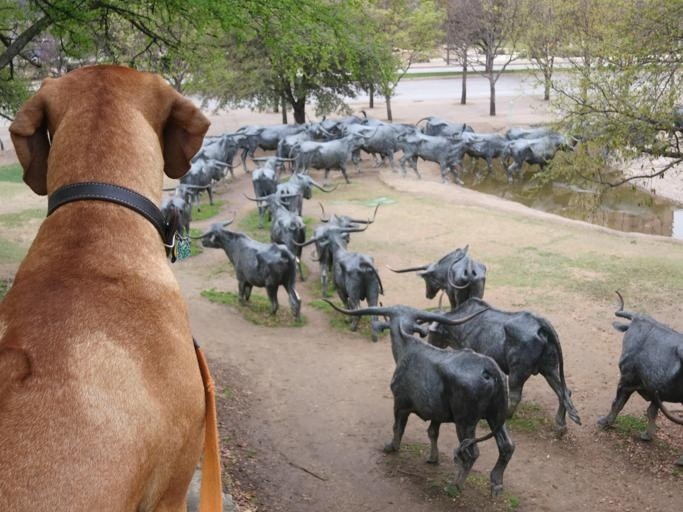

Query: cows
<box><xmin>243</xmin><ymin>192</ymin><xmax>307</xmax><ymax>271</ymax></box>
<box><xmin>177</xmin><ymin>111</ymin><xmax>593</xmax><ymax>229</ymax></box>
<box><xmin>384</xmin><ymin>244</ymin><xmax>487</xmax><ymax>311</ymax></box>
<box><xmin>161</xmin><ymin>184</ymin><xmax>210</xmax><ymax>243</ymax></box>
<box><xmin>186</xmin><ymin>219</ymin><xmax>306</xmax><ymax>323</ymax></box>
<box><xmin>321</xmin><ymin>297</ymin><xmax>515</xmax><ymax>500</ymax></box>
<box><xmin>291</xmin><ymin>219</ymin><xmax>383</xmax><ymax>342</ymax></box>
<box><xmin>597</xmin><ymin>291</ymin><xmax>683</xmax><ymax>441</ymax></box>
<box><xmin>311</xmin><ymin>202</ymin><xmax>377</xmax><ymax>298</ymax></box>
<box><xmin>379</xmin><ymin>302</ymin><xmax>582</xmax><ymax>439</ymax></box>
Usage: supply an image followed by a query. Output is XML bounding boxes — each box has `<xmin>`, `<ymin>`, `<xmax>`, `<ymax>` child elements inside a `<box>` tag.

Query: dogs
<box><xmin>0</xmin><ymin>64</ymin><xmax>210</xmax><ymax>512</ymax></box>
